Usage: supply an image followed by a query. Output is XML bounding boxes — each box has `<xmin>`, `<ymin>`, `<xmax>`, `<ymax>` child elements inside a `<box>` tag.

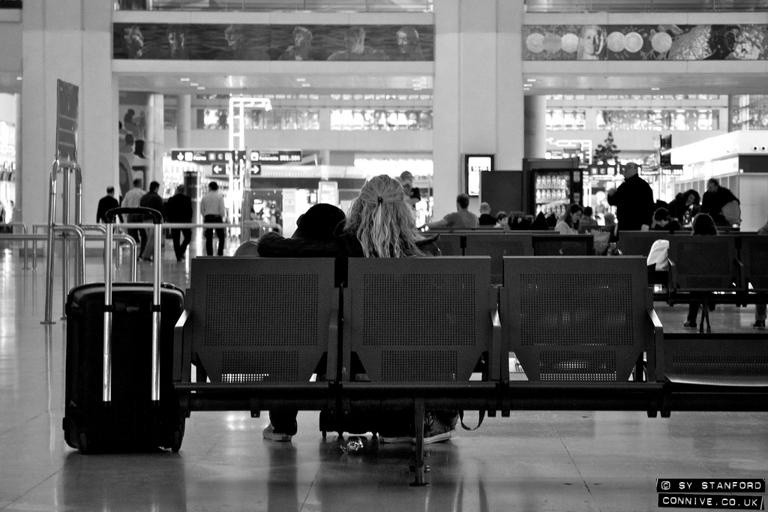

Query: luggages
<box><xmin>62</xmin><ymin>208</ymin><xmax>185</xmax><ymax>454</ymax></box>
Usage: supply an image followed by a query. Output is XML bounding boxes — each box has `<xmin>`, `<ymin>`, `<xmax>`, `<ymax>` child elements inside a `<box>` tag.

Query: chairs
<box><xmin>169</xmin><ymin>226</ymin><xmax>767</xmax><ymax>486</ymax></box>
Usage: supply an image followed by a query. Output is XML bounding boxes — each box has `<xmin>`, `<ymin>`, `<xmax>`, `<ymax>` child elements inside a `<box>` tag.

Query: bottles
<box><xmin>534</xmin><ymin>174</ymin><xmax>567</xmax><ymax>217</ymax></box>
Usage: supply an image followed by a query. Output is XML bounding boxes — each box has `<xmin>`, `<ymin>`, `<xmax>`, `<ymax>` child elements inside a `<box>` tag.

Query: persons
<box><xmin>256</xmin><ymin>161</ymin><xmax>741</xmax><ymax>443</ymax></box>
<box><xmin>95</xmin><ymin>178</ymin><xmax>193</xmax><ymax>263</ymax></box>
<box><xmin>201</xmin><ymin>181</ymin><xmax>225</xmax><ymax>257</ymax></box>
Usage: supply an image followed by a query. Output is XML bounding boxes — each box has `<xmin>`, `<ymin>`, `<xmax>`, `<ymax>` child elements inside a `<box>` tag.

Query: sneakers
<box><xmin>262</xmin><ymin>423</ymin><xmax>291</xmax><ymax>442</ymax></box>
<box><xmin>425</xmin><ymin>410</ymin><xmax>451</xmax><ymax>442</ymax></box>
<box><xmin>683</xmin><ymin>322</ymin><xmax>697</xmax><ymax>329</ymax></box>
<box><xmin>753</xmin><ymin>320</ymin><xmax>765</xmax><ymax>328</ymax></box>
